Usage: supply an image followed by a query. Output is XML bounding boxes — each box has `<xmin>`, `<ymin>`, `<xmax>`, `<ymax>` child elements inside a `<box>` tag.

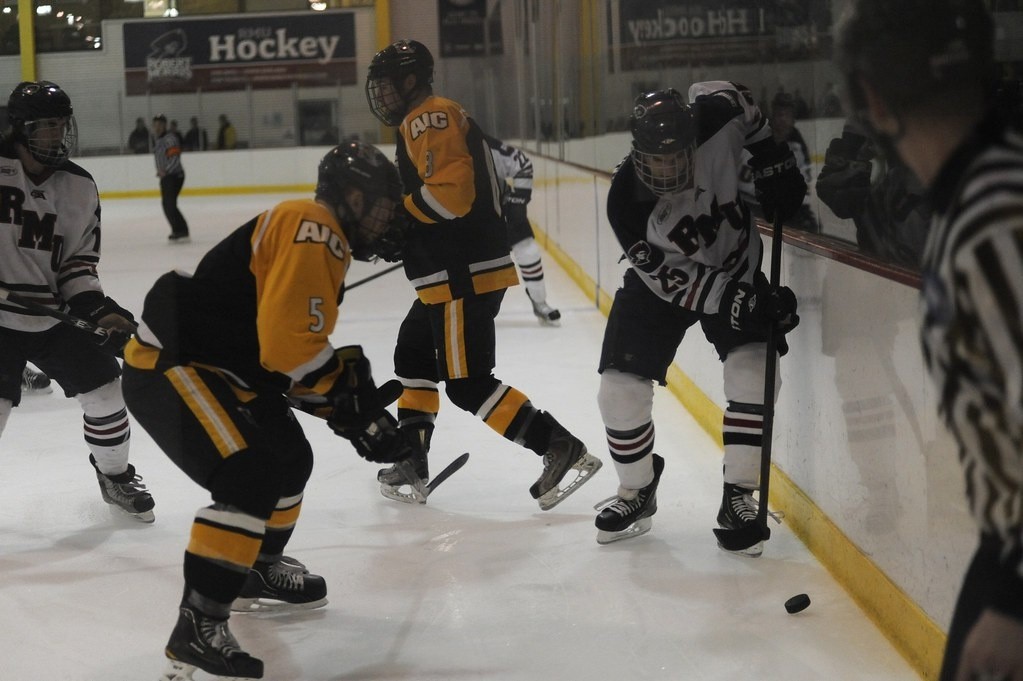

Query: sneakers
<box><xmin>592</xmin><ymin>452</ymin><xmax>664</xmax><ymax>545</ymax></box>
<box><xmin>89</xmin><ymin>453</ymin><xmax>156</xmax><ymax>522</ymax></box>
<box><xmin>377</xmin><ymin>420</ymin><xmax>435</xmax><ymax>505</ymax></box>
<box><xmin>23</xmin><ymin>366</ymin><xmax>53</xmax><ymax>394</ymax></box>
<box><xmin>522</xmin><ymin>409</ymin><xmax>603</xmax><ymax>511</ymax></box>
<box><xmin>161</xmin><ymin>604</ymin><xmax>264</xmax><ymax>681</ymax></box>
<box><xmin>230</xmin><ymin>555</ymin><xmax>329</xmax><ymax>611</ymax></box>
<box><xmin>716</xmin><ymin>464</ymin><xmax>764</xmax><ymax>558</ymax></box>
<box><xmin>168</xmin><ymin>226</ymin><xmax>190</xmax><ymax>244</ymax></box>
<box><xmin>524</xmin><ymin>286</ymin><xmax>561</xmax><ymax>329</ymax></box>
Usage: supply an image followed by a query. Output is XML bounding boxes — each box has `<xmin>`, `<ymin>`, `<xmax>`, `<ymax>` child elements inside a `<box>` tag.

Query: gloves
<box><xmin>815</xmin><ymin>139</ymin><xmax>874</xmax><ymax>219</ymax></box>
<box><xmin>748</xmin><ymin>141</ymin><xmax>807</xmax><ymax>223</ymax></box>
<box><xmin>372</xmin><ymin>202</ymin><xmax>419</xmax><ymax>261</ymax></box>
<box><xmin>719</xmin><ymin>272</ymin><xmax>799</xmax><ymax>356</ymax></box>
<box><xmin>328</xmin><ymin>344</ymin><xmax>415</xmax><ymax>464</ymax></box>
<box><xmin>72</xmin><ymin>293</ymin><xmax>138</xmax><ymax>352</ymax></box>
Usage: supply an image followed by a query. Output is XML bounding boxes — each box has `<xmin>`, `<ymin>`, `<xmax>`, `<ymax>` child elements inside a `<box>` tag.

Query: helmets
<box><xmin>314</xmin><ymin>142</ymin><xmax>405</xmax><ymax>262</ymax></box>
<box><xmin>628</xmin><ymin>86</ymin><xmax>696</xmax><ymax>199</ymax></box>
<box><xmin>364</xmin><ymin>38</ymin><xmax>433</xmax><ymax>127</ymax></box>
<box><xmin>7</xmin><ymin>80</ymin><xmax>78</xmax><ymax>164</ymax></box>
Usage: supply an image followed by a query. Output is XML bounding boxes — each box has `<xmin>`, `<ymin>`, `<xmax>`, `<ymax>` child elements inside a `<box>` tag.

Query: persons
<box><xmin>216</xmin><ymin>114</ymin><xmax>237</xmax><ymax>150</ymax></box>
<box><xmin>152</xmin><ymin>113</ymin><xmax>189</xmax><ymax>240</ymax></box>
<box><xmin>0</xmin><ymin>80</ymin><xmax>156</xmax><ymax>514</ymax></box>
<box><xmin>364</xmin><ymin>37</ymin><xmax>587</xmax><ymax>500</ymax></box>
<box><xmin>738</xmin><ymin>94</ymin><xmax>929</xmax><ymax>273</ymax></box>
<box><xmin>595</xmin><ymin>80</ymin><xmax>808</xmax><ymax>541</ymax></box>
<box><xmin>128</xmin><ymin>116</ymin><xmax>207</xmax><ymax>154</ymax></box>
<box><xmin>121</xmin><ymin>142</ymin><xmax>415</xmax><ymax>678</ymax></box>
<box><xmin>831</xmin><ymin>0</ymin><xmax>1023</xmax><ymax>681</ymax></box>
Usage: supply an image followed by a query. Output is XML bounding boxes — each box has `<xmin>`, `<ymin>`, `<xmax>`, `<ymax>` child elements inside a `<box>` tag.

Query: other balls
<box><xmin>784</xmin><ymin>593</ymin><xmax>811</xmax><ymax>614</ymax></box>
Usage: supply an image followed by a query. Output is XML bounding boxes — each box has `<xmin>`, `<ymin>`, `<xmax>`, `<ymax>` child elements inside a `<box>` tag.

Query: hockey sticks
<box><xmin>712</xmin><ymin>217</ymin><xmax>785</xmax><ymax>551</ymax></box>
<box><xmin>0</xmin><ymin>287</ymin><xmax>404</xmax><ymax>429</ymax></box>
<box><xmin>374</xmin><ymin>411</ymin><xmax>470</xmax><ymax>498</ymax></box>
<box><xmin>345</xmin><ymin>262</ymin><xmax>403</xmax><ymax>291</ymax></box>
